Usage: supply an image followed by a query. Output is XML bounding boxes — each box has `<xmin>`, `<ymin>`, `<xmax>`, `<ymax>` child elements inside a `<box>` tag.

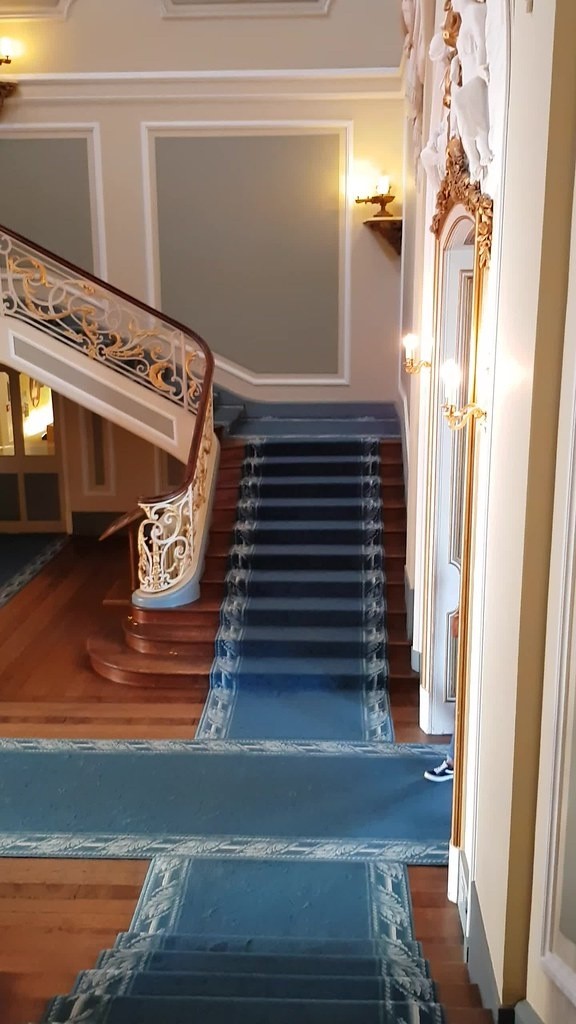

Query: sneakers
<box><xmin>424</xmin><ymin>761</ymin><xmax>455</xmax><ymax>782</ymax></box>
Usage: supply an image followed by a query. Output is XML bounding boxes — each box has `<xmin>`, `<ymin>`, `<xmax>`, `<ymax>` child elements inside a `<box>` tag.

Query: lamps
<box><xmin>0</xmin><ymin>36</ymin><xmax>12</xmax><ymax>65</ymax></box>
<box><xmin>356</xmin><ymin>175</ymin><xmax>396</xmax><ymax>217</ymax></box>
